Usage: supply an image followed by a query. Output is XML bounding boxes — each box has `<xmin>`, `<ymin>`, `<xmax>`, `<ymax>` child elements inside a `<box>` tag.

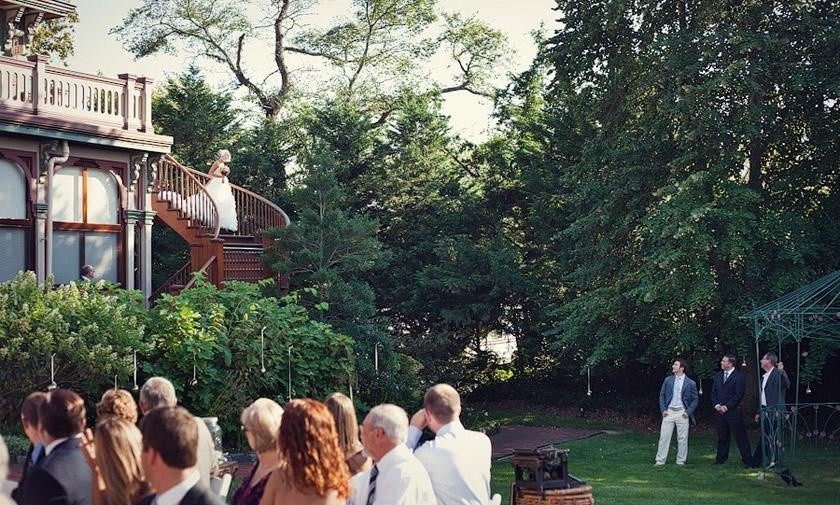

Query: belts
<box><xmin>668</xmin><ymin>406</ymin><xmax>686</xmax><ymax>410</ymax></box>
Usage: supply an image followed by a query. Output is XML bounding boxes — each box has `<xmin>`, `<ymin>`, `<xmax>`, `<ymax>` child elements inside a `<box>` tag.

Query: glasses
<box><xmin>240</xmin><ymin>424</ymin><xmax>246</xmax><ymax>432</ymax></box>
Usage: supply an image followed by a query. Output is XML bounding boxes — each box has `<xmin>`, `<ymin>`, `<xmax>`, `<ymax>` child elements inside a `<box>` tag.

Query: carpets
<box><xmin>490</xmin><ymin>424</ymin><xmax>604</xmax><ymax>459</ymax></box>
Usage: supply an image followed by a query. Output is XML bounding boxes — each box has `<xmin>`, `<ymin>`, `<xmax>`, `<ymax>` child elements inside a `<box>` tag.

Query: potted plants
<box><xmin>27</xmin><ymin>10</ymin><xmax>80</xmax><ymax>66</ymax></box>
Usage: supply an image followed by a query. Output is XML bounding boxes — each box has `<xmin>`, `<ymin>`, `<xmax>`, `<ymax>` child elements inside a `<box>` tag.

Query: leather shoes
<box><xmin>711</xmin><ymin>460</ymin><xmax>778</xmax><ymax>469</ymax></box>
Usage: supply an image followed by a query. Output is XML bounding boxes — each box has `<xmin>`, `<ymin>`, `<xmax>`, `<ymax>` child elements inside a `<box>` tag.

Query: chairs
<box><xmin>349</xmin><ymin>470</ymin><xmax>363</xmax><ymax>501</ymax></box>
<box><xmin>211</xmin><ymin>476</ymin><xmax>221</xmax><ymax>492</ymax></box>
<box><xmin>491</xmin><ymin>492</ymin><xmax>502</xmax><ymax>503</ymax></box>
<box><xmin>217</xmin><ymin>473</ymin><xmax>233</xmax><ymax>501</ymax></box>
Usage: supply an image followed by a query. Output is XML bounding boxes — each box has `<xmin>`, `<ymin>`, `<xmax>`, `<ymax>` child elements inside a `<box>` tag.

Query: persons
<box><xmin>80</xmin><ymin>265</ymin><xmax>94</xmax><ymax>282</ymax></box>
<box><xmin>745</xmin><ymin>351</ymin><xmax>791</xmax><ymax>468</ymax></box>
<box><xmin>157</xmin><ymin>149</ymin><xmax>239</xmax><ymax>233</ymax></box>
<box><xmin>711</xmin><ymin>354</ymin><xmax>752</xmax><ymax>465</ymax></box>
<box><xmin>654</xmin><ymin>358</ymin><xmax>699</xmax><ymax>467</ymax></box>
<box><xmin>1</xmin><ymin>375</ymin><xmax>492</xmax><ymax>505</ymax></box>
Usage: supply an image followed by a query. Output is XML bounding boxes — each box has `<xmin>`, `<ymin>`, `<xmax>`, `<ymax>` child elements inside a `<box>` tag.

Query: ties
<box><xmin>724</xmin><ymin>372</ymin><xmax>728</xmax><ymax>383</ymax></box>
<box><xmin>367</xmin><ymin>464</ymin><xmax>378</xmax><ymax>504</ymax></box>
<box><xmin>677</xmin><ymin>379</ymin><xmax>681</xmax><ymax>391</ymax></box>
